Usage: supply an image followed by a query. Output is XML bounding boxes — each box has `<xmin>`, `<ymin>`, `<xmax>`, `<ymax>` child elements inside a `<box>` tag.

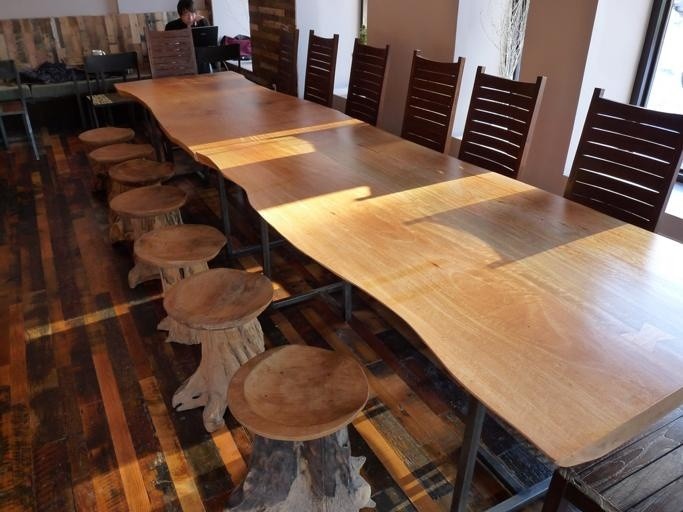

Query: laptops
<box><xmin>191</xmin><ymin>26</ymin><xmax>218</xmax><ymax>47</ymax></box>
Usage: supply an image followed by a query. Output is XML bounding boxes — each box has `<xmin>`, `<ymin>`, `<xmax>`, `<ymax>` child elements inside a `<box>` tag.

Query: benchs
<box><xmin>541</xmin><ymin>404</ymin><xmax>683</xmax><ymax>511</ymax></box>
<box><xmin>0</xmin><ymin>8</ymin><xmax>209</xmax><ymax>98</ymax></box>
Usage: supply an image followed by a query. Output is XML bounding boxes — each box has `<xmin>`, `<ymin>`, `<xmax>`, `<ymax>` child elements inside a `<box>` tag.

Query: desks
<box><xmin>113</xmin><ymin>70</ymin><xmax>683</xmax><ymax>511</ymax></box>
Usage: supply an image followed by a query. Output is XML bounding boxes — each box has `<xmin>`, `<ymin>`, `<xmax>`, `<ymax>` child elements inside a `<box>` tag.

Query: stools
<box><xmin>162</xmin><ymin>265</ymin><xmax>275</xmax><ymax>436</ymax></box>
<box><xmin>222</xmin><ymin>343</ymin><xmax>377</xmax><ymax>510</ymax></box>
<box><xmin>87</xmin><ymin>141</ymin><xmax>159</xmax><ymax>179</ymax></box>
<box><xmin>79</xmin><ymin>125</ymin><xmax>135</xmax><ymax>147</ymax></box>
<box><xmin>134</xmin><ymin>223</ymin><xmax>228</xmax><ymax>347</ymax></box>
<box><xmin>108</xmin><ymin>159</ymin><xmax>177</xmax><ymax>242</ymax></box>
<box><xmin>109</xmin><ymin>184</ymin><xmax>191</xmax><ymax>290</ymax></box>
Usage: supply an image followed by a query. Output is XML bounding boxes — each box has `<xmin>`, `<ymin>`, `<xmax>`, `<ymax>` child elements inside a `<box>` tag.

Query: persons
<box><xmin>165</xmin><ymin>1</ymin><xmax>222</xmax><ymax>75</ymax></box>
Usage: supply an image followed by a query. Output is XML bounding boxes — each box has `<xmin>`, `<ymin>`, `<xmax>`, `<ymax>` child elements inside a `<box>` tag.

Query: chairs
<box><xmin>269</xmin><ymin>25</ymin><xmax>302</xmax><ymax>99</ymax></box>
<box><xmin>562</xmin><ymin>87</ymin><xmax>682</xmax><ymax>235</ymax></box>
<box><xmin>85</xmin><ymin>51</ymin><xmax>152</xmax><ymax>134</ymax></box>
<box><xmin>143</xmin><ymin>23</ymin><xmax>241</xmax><ymax>166</ymax></box>
<box><xmin>0</xmin><ymin>59</ymin><xmax>40</xmax><ymax>162</ymax></box>
<box><xmin>302</xmin><ymin>30</ymin><xmax>342</xmax><ymax>109</ymax></box>
<box><xmin>343</xmin><ymin>37</ymin><xmax>393</xmax><ymax>128</ymax></box>
<box><xmin>455</xmin><ymin>64</ymin><xmax>549</xmax><ymax>182</ymax></box>
<box><xmin>398</xmin><ymin>48</ymin><xmax>467</xmax><ymax>155</ymax></box>
